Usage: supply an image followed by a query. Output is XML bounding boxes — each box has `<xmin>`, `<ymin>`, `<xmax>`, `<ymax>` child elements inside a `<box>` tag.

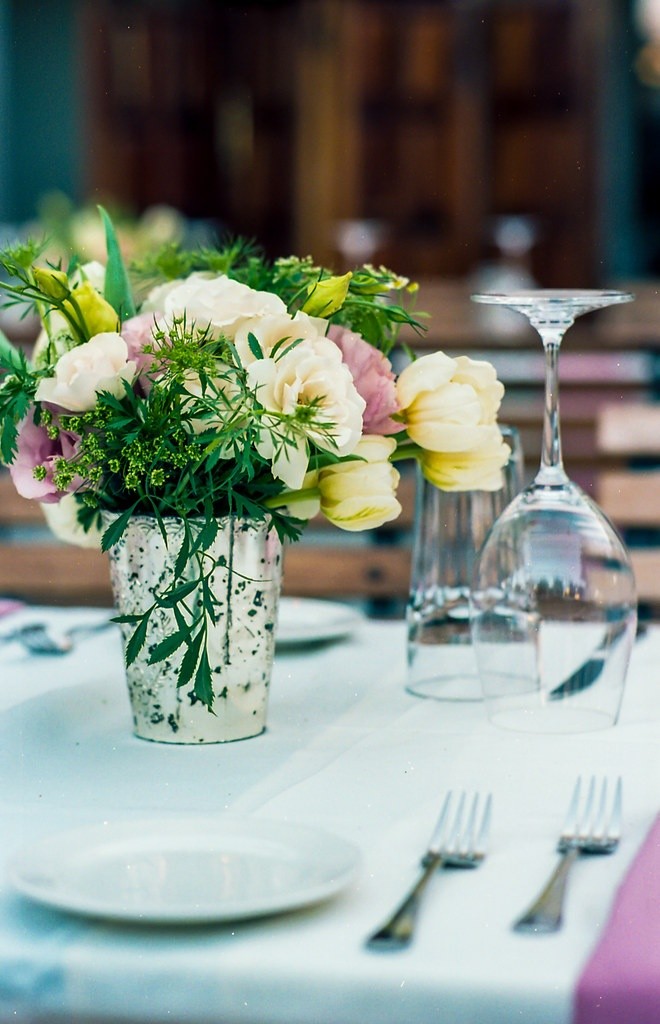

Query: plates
<box><xmin>6</xmin><ymin>813</ymin><xmax>361</xmax><ymax>925</ymax></box>
<box><xmin>274</xmin><ymin>598</ymin><xmax>365</xmax><ymax>650</ymax></box>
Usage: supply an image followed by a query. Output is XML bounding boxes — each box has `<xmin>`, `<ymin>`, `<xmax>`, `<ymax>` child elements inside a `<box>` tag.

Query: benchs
<box><xmin>0</xmin><ymin>282</ymin><xmax>660</xmax><ymax>613</ymax></box>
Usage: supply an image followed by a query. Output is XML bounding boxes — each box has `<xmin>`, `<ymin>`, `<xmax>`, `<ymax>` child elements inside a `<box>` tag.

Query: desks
<box><xmin>0</xmin><ymin>599</ymin><xmax>660</xmax><ymax>1024</ymax></box>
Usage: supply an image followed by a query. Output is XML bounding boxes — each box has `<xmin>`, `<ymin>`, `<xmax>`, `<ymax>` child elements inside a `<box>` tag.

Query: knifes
<box><xmin>548</xmin><ymin>622</ymin><xmax>628</xmax><ymax>697</ymax></box>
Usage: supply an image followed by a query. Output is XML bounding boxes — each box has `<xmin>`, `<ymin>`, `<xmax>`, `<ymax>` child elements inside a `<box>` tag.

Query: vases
<box><xmin>98</xmin><ymin>508</ymin><xmax>283</xmax><ymax>746</ymax></box>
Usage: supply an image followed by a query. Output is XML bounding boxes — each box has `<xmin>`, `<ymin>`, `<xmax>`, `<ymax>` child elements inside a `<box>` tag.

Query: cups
<box><xmin>398</xmin><ymin>422</ymin><xmax>543</xmax><ymax>703</ymax></box>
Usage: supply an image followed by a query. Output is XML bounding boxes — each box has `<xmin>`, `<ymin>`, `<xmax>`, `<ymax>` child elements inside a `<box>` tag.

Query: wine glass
<box><xmin>468</xmin><ymin>291</ymin><xmax>638</xmax><ymax>734</ymax></box>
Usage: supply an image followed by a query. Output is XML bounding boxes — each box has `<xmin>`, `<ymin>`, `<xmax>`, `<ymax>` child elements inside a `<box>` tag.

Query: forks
<box><xmin>363</xmin><ymin>790</ymin><xmax>494</xmax><ymax>952</ymax></box>
<box><xmin>18</xmin><ymin>618</ymin><xmax>119</xmax><ymax>654</ymax></box>
<box><xmin>513</xmin><ymin>772</ymin><xmax>624</xmax><ymax>933</ymax></box>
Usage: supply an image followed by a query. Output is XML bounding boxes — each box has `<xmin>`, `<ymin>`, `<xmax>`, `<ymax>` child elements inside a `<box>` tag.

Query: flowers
<box><xmin>0</xmin><ymin>202</ymin><xmax>513</xmax><ymax>707</ymax></box>
